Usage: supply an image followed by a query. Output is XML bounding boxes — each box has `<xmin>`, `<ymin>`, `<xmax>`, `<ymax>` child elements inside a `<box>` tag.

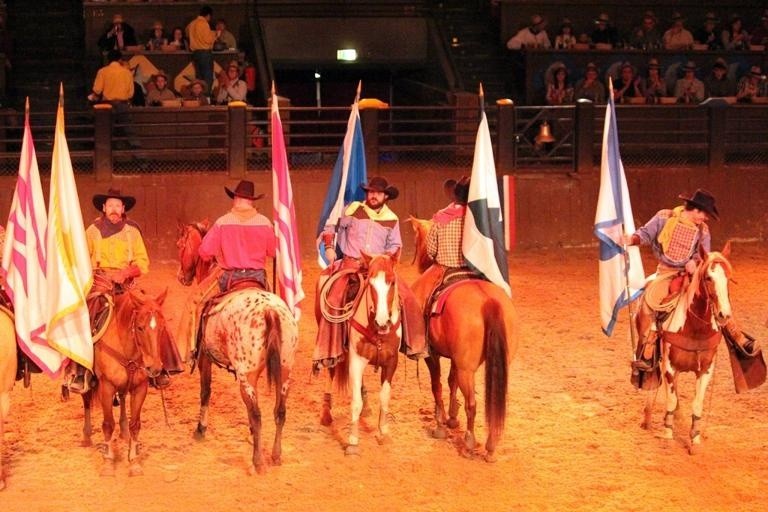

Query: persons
<box><xmin>70</xmin><ymin>188</ymin><xmax>170</xmax><ymax>390</ymax></box>
<box><xmin>178</xmin><ymin>181</ymin><xmax>276</xmax><ymax>362</ymax></box>
<box><xmin>507</xmin><ymin>10</ymin><xmax>768</xmax><ymax>106</ymax></box>
<box><xmin>316</xmin><ymin>177</ymin><xmax>428</xmax><ymax>368</ymax></box>
<box><xmin>409</xmin><ymin>177</ymin><xmax>487</xmax><ymax>314</ymax></box>
<box><xmin>88</xmin><ymin>7</ymin><xmax>264</xmax><ymax>147</ymax></box>
<box><xmin>620</xmin><ymin>188</ymin><xmax>759</xmax><ymax>369</ymax></box>
<box><xmin>0</xmin><ymin>0</ymin><xmax>13</xmax><ymax>69</ymax></box>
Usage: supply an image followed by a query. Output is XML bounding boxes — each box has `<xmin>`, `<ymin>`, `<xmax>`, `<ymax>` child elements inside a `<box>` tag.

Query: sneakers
<box><xmin>637</xmin><ymin>358</ymin><xmax>652</xmax><ymax>369</ymax></box>
<box><xmin>739</xmin><ymin>339</ymin><xmax>758</xmax><ymax>360</ymax></box>
<box><xmin>151</xmin><ymin>376</ymin><xmax>169</xmax><ymax>388</ymax></box>
<box><xmin>72</xmin><ymin>374</ymin><xmax>84</xmax><ymax>392</ymax></box>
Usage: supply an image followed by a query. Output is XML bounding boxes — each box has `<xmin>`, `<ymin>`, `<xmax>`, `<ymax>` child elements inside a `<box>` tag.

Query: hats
<box><xmin>112</xmin><ymin>13</ymin><xmax>240</xmax><ymax>94</ymax></box>
<box><xmin>678</xmin><ymin>188</ymin><xmax>721</xmax><ymax>223</ymax></box>
<box><xmin>443</xmin><ymin>174</ymin><xmax>470</xmax><ymax>196</ymax></box>
<box><xmin>224</xmin><ymin>180</ymin><xmax>266</xmax><ymax>201</ymax></box>
<box><xmin>529</xmin><ymin>12</ymin><xmax>767</xmax><ymax>83</ymax></box>
<box><xmin>92</xmin><ymin>188</ymin><xmax>136</xmax><ymax>210</ymax></box>
<box><xmin>359</xmin><ymin>176</ymin><xmax>399</xmax><ymax>200</ymax></box>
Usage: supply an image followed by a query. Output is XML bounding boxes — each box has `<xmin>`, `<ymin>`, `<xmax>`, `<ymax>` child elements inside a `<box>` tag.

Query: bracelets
<box><xmin>124</xmin><ymin>266</ymin><xmax>141</xmax><ymax>279</ymax></box>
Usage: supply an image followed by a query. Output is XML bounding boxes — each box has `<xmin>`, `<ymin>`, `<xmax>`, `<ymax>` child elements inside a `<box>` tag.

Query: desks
<box><xmin>93</xmin><ymin>43</ymin><xmax>248</xmax><ymax>164</ymax></box>
<box><xmin>523</xmin><ymin>44</ymin><xmax>768</xmax><ymax>161</ymax></box>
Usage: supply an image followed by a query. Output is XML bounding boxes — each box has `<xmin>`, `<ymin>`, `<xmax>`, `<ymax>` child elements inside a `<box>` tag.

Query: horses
<box><xmin>0</xmin><ymin>306</ymin><xmax>19</xmax><ymax>492</ymax></box>
<box><xmin>639</xmin><ymin>240</ymin><xmax>734</xmax><ymax>457</ymax></box>
<box><xmin>81</xmin><ymin>285</ymin><xmax>168</xmax><ymax>478</ymax></box>
<box><xmin>314</xmin><ymin>246</ymin><xmax>403</xmax><ymax>457</ymax></box>
<box><xmin>403</xmin><ymin>213</ymin><xmax>519</xmax><ymax>463</ymax></box>
<box><xmin>176</xmin><ymin>215</ymin><xmax>298</xmax><ymax>477</ymax></box>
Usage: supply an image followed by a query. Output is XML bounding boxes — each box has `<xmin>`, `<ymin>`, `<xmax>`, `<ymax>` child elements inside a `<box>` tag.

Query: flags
<box><xmin>593</xmin><ymin>97</ymin><xmax>646</xmax><ymax>338</ymax></box>
<box><xmin>46</xmin><ymin>101</ymin><xmax>93</xmax><ymax>375</ymax></box>
<box><xmin>462</xmin><ymin>110</ymin><xmax>513</xmax><ymax>298</ymax></box>
<box><xmin>272</xmin><ymin>94</ymin><xmax>305</xmax><ymax>322</ymax></box>
<box><xmin>315</xmin><ymin>104</ymin><xmax>368</xmax><ymax>271</ymax></box>
<box><xmin>1</xmin><ymin>113</ymin><xmax>71</xmax><ymax>380</ymax></box>
